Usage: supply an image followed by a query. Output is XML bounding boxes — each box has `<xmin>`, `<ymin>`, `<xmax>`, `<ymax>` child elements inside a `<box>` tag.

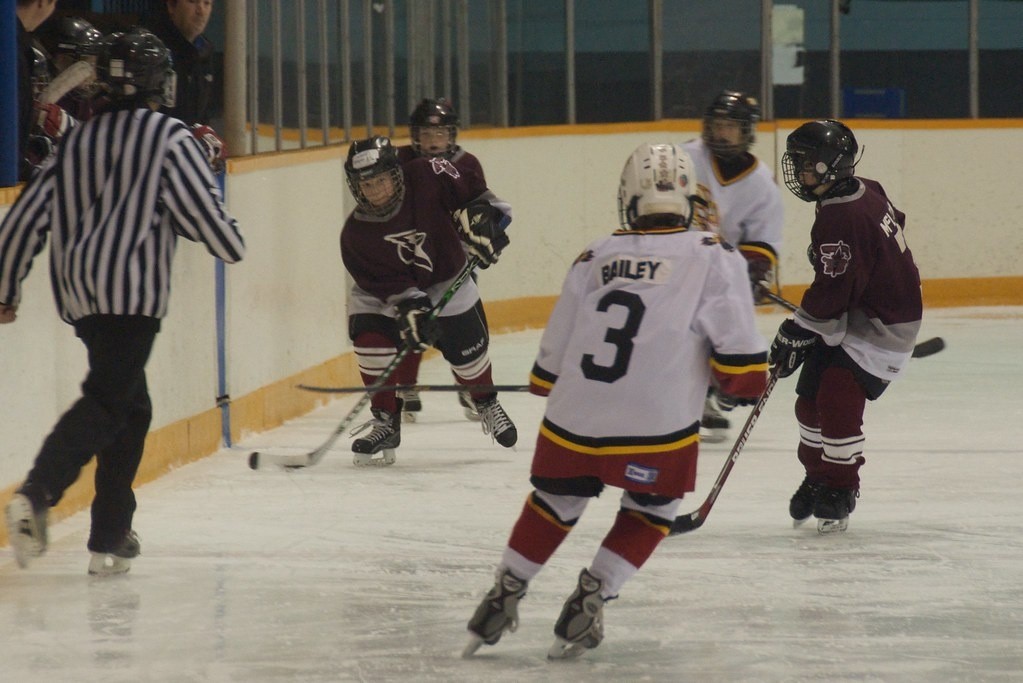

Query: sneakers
<box><xmin>348</xmin><ymin>397</ymin><xmax>404</xmax><ymax>469</ymax></box>
<box><xmin>394</xmin><ymin>384</ymin><xmax>422</xmax><ymax>424</ymax></box>
<box><xmin>788</xmin><ymin>472</ymin><xmax>860</xmax><ymax>534</ymax></box>
<box><xmin>470</xmin><ymin>391</ymin><xmax>519</xmax><ymax>455</ymax></box>
<box><xmin>698</xmin><ymin>384</ymin><xmax>732</xmax><ymax>442</ymax></box>
<box><xmin>4</xmin><ymin>482</ymin><xmax>50</xmax><ymax>568</ymax></box>
<box><xmin>544</xmin><ymin>567</ymin><xmax>620</xmax><ymax>660</ymax></box>
<box><xmin>460</xmin><ymin>561</ymin><xmax>531</xmax><ymax>658</ymax></box>
<box><xmin>455</xmin><ymin>385</ymin><xmax>481</xmax><ymax>421</ymax></box>
<box><xmin>87</xmin><ymin>528</ymin><xmax>144</xmax><ymax>576</ymax></box>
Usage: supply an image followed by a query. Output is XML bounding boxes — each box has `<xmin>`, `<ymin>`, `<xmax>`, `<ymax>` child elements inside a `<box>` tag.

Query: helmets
<box><xmin>409</xmin><ymin>97</ymin><xmax>459</xmax><ymax>159</ymax></box>
<box><xmin>343</xmin><ymin>134</ymin><xmax>407</xmax><ymax>223</ymax></box>
<box><xmin>46</xmin><ymin>16</ymin><xmax>110</xmax><ymax>102</ymax></box>
<box><xmin>96</xmin><ymin>28</ymin><xmax>172</xmax><ymax>112</ymax></box>
<box><xmin>701</xmin><ymin>91</ymin><xmax>761</xmax><ymax>162</ymax></box>
<box><xmin>781</xmin><ymin>119</ymin><xmax>866</xmax><ymax>202</ymax></box>
<box><xmin>616</xmin><ymin>140</ymin><xmax>707</xmax><ymax>234</ymax></box>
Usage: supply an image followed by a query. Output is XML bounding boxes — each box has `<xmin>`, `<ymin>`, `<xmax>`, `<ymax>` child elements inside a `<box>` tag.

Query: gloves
<box><xmin>767</xmin><ymin>318</ymin><xmax>819</xmax><ymax>378</ymax></box>
<box><xmin>388</xmin><ymin>289</ymin><xmax>440</xmax><ymax>355</ymax></box>
<box><xmin>455</xmin><ymin>202</ymin><xmax>503</xmax><ymax>270</ymax></box>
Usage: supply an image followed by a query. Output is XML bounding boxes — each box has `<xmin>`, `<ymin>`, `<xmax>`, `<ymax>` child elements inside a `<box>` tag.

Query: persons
<box><xmin>768</xmin><ymin>119</ymin><xmax>923</xmax><ymax>520</ymax></box>
<box><xmin>677</xmin><ymin>90</ymin><xmax>787</xmax><ymax>428</ymax></box>
<box><xmin>394</xmin><ymin>97</ymin><xmax>512</xmax><ymax>412</ymax></box>
<box><xmin>17</xmin><ymin>0</ymin><xmax>226</xmax><ymax>182</ymax></box>
<box><xmin>0</xmin><ymin>27</ymin><xmax>248</xmax><ymax>560</ymax></box>
<box><xmin>340</xmin><ymin>134</ymin><xmax>518</xmax><ymax>455</ymax></box>
<box><xmin>467</xmin><ymin>143</ymin><xmax>770</xmax><ymax>649</ymax></box>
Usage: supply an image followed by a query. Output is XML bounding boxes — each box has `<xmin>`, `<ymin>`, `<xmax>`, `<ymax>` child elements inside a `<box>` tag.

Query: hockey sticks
<box><xmin>246</xmin><ymin>253</ymin><xmax>483</xmax><ymax>473</ymax></box>
<box><xmin>297</xmin><ymin>382</ymin><xmax>530</xmax><ymax>393</ymax></box>
<box><xmin>672</xmin><ymin>360</ymin><xmax>788</xmax><ymax>535</ymax></box>
<box><xmin>749</xmin><ymin>287</ymin><xmax>947</xmax><ymax>360</ymax></box>
